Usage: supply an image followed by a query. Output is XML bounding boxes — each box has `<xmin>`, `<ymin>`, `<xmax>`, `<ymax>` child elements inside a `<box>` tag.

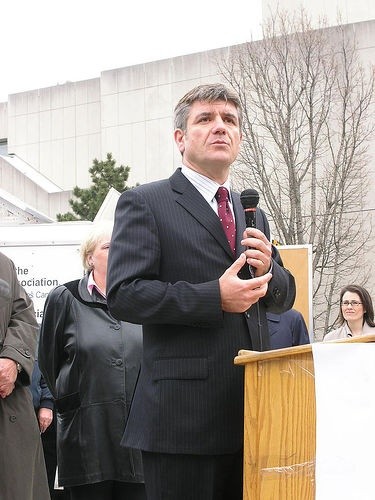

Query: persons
<box><xmin>0</xmin><ymin>252</ymin><xmax>57</xmax><ymax>500</ymax></box>
<box><xmin>37</xmin><ymin>232</ymin><xmax>147</xmax><ymax>500</ymax></box>
<box><xmin>324</xmin><ymin>285</ymin><xmax>375</xmax><ymax>340</ymax></box>
<box><xmin>266</xmin><ymin>309</ymin><xmax>310</xmax><ymax>351</ymax></box>
<box><xmin>106</xmin><ymin>84</ymin><xmax>296</xmax><ymax>500</ymax></box>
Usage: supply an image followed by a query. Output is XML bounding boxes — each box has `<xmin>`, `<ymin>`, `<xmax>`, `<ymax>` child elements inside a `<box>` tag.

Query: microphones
<box><xmin>240</xmin><ymin>189</ymin><xmax>259</xmax><ymax>257</ymax></box>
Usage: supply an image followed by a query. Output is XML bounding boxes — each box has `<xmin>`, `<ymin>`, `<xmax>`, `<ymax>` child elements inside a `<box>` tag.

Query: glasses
<box><xmin>342</xmin><ymin>300</ymin><xmax>362</xmax><ymax>307</ymax></box>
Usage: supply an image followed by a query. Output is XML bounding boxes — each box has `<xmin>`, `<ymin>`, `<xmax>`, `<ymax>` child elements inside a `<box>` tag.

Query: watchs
<box><xmin>17</xmin><ymin>362</ymin><xmax>23</xmax><ymax>373</ymax></box>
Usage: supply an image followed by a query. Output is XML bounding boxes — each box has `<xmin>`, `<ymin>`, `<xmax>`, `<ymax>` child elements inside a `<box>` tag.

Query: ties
<box><xmin>214</xmin><ymin>187</ymin><xmax>237</xmax><ymax>258</ymax></box>
